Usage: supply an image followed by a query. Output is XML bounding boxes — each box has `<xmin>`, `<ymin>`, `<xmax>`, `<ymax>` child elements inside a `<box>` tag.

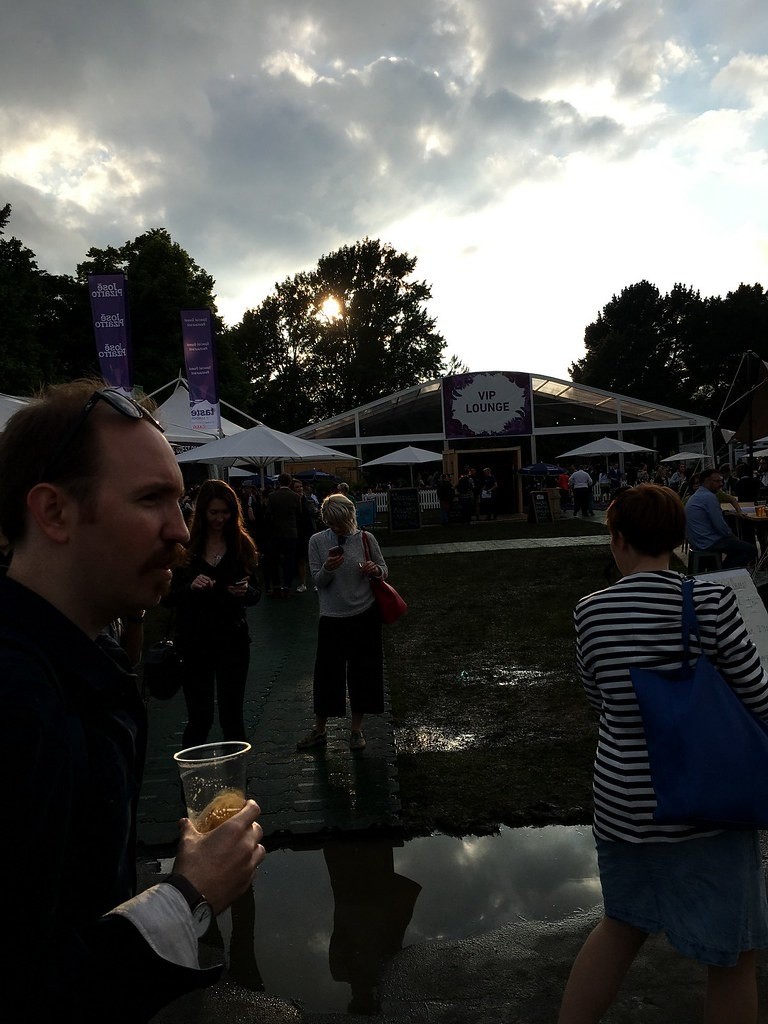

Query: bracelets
<box><xmin>126</xmin><ymin>615</ymin><xmax>144</xmax><ymax>624</ymax></box>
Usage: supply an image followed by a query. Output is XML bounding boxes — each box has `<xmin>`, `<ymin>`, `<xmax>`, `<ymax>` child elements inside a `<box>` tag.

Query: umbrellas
<box><xmin>554</xmin><ymin>435</ymin><xmax>660</xmax><ymax>473</ymax></box>
<box><xmin>659</xmin><ymin>451</ymin><xmax>712</xmax><ymax>466</ymax></box>
<box><xmin>752</xmin><ymin>435</ymin><xmax>768</xmax><ymax>443</ymax></box>
<box><xmin>518</xmin><ymin>460</ymin><xmax>565</xmax><ymax>491</ymax></box>
<box><xmin>175</xmin><ymin>423</ymin><xmax>362</xmax><ymax>491</ymax></box>
<box><xmin>741</xmin><ymin>448</ymin><xmax>768</xmax><ymax>457</ymax></box>
<box><xmin>357</xmin><ymin>444</ymin><xmax>444</xmax><ymax>487</ymax></box>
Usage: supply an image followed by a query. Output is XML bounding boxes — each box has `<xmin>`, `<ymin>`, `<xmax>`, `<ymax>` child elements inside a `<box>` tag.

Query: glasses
<box><xmin>40</xmin><ymin>386</ymin><xmax>166</xmax><ymax>484</ymax></box>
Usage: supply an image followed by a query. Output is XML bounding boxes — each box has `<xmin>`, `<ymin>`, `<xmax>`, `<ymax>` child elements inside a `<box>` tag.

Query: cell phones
<box><xmin>329</xmin><ymin>546</ymin><xmax>340</xmax><ymax>557</ymax></box>
<box><xmin>236</xmin><ymin>581</ymin><xmax>247</xmax><ymax>587</ymax></box>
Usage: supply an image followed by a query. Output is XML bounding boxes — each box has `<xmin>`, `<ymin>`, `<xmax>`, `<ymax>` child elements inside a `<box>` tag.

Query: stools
<box><xmin>687</xmin><ymin>547</ymin><xmax>722</xmax><ymax>575</ymax></box>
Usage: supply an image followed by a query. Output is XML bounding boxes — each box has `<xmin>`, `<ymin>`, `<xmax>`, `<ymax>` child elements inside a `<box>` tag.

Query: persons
<box><xmin>169</xmin><ymin>477</ymin><xmax>262</xmax><ymax>804</ymax></box>
<box><xmin>102</xmin><ymin>607</ymin><xmax>146</xmax><ymax>667</ymax></box>
<box><xmin>291</xmin><ymin>477</ymin><xmax>314</xmax><ymax>577</ymax></box>
<box><xmin>336</xmin><ymin>481</ymin><xmax>357</xmax><ymax>509</ymax></box>
<box><xmin>554</xmin><ymin>455</ymin><xmax>767</xmax><ymax>516</ymax></box>
<box><xmin>684</xmin><ymin>469</ymin><xmax>758</xmax><ymax>569</ymax></box>
<box><xmin>475</xmin><ymin>467</ymin><xmax>498</xmax><ymax>520</ymax></box>
<box><xmin>437</xmin><ymin>474</ymin><xmax>455</xmax><ymax>525</ymax></box>
<box><xmin>451</xmin><ymin>467</ymin><xmax>480</xmax><ymax>526</ymax></box>
<box><xmin>264</xmin><ymin>472</ymin><xmax>303</xmax><ymax>596</ymax></box>
<box><xmin>296</xmin><ymin>492</ymin><xmax>391</xmax><ymax>750</ymax></box>
<box><xmin>0</xmin><ymin>374</ymin><xmax>266</xmax><ymax>1023</ymax></box>
<box><xmin>559</xmin><ymin>482</ymin><xmax>768</xmax><ymax>1023</ymax></box>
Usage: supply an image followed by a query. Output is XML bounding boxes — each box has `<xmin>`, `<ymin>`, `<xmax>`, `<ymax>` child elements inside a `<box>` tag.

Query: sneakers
<box><xmin>297</xmin><ymin>727</ymin><xmax>328</xmax><ymax>750</ymax></box>
<box><xmin>349</xmin><ymin>730</ymin><xmax>366</xmax><ymax>750</ymax></box>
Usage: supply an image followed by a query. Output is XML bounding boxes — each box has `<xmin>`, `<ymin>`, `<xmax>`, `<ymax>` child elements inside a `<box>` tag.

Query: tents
<box><xmin>288</xmin><ymin>370</ymin><xmax>720</xmax><ymax>489</ymax></box>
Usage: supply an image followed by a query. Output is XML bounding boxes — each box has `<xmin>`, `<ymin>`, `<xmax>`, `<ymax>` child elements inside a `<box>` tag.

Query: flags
<box><xmin>181</xmin><ymin>307</ymin><xmax>223</xmax><ymax>439</ymax></box>
<box><xmin>87</xmin><ymin>272</ymin><xmax>133</xmax><ymax>392</ymax></box>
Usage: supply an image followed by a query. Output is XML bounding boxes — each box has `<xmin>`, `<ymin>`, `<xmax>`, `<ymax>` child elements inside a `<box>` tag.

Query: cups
<box><xmin>755</xmin><ymin>506</ymin><xmax>763</xmax><ymax>517</ymax></box>
<box><xmin>173</xmin><ymin>741</ymin><xmax>252</xmax><ymax>835</ymax></box>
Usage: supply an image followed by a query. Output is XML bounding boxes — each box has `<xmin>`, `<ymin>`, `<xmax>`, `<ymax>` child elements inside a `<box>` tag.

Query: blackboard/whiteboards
<box><xmin>386</xmin><ymin>487</ymin><xmax>422</xmax><ymax>533</ymax></box>
<box><xmin>526</xmin><ymin>490</ymin><xmax>555</xmax><ymax>526</ymax></box>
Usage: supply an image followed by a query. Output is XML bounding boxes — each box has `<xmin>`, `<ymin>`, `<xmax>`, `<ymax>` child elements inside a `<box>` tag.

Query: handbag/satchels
<box><xmin>362</xmin><ymin>530</ymin><xmax>408</xmax><ymax>624</ymax></box>
<box><xmin>146</xmin><ymin>640</ymin><xmax>185</xmax><ymax>701</ymax></box>
<box><xmin>629</xmin><ymin>578</ymin><xmax>767</xmax><ymax>830</ymax></box>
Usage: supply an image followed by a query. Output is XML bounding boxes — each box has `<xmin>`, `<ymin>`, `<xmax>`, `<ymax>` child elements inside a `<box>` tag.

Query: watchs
<box><xmin>162</xmin><ymin>872</ymin><xmax>213</xmax><ymax>939</ymax></box>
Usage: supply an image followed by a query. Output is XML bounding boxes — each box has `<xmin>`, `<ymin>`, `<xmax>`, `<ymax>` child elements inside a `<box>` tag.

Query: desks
<box><xmin>721</xmin><ymin>503</ymin><xmax>768</xmax><ymax>539</ymax></box>
